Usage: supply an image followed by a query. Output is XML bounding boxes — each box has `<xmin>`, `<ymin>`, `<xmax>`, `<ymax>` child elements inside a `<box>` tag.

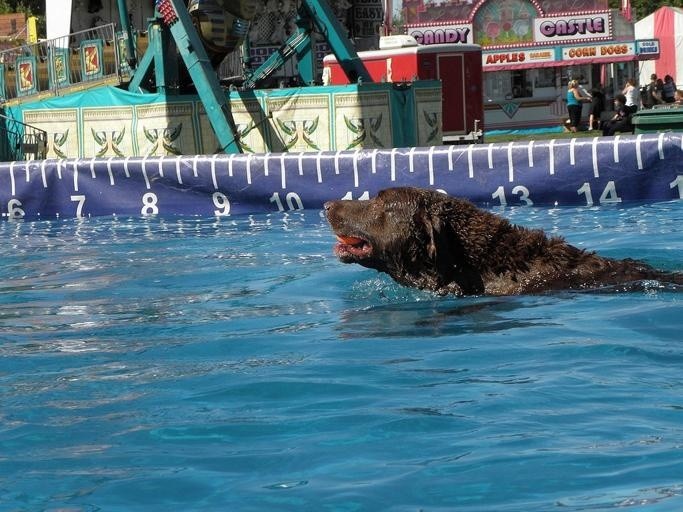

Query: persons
<box><xmin>566</xmin><ymin>73</ymin><xmax>683</xmax><ymax>136</ymax></box>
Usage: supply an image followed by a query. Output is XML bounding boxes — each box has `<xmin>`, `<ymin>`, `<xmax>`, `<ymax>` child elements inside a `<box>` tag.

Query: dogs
<box><xmin>324</xmin><ymin>184</ymin><xmax>683</xmax><ymax>340</ymax></box>
